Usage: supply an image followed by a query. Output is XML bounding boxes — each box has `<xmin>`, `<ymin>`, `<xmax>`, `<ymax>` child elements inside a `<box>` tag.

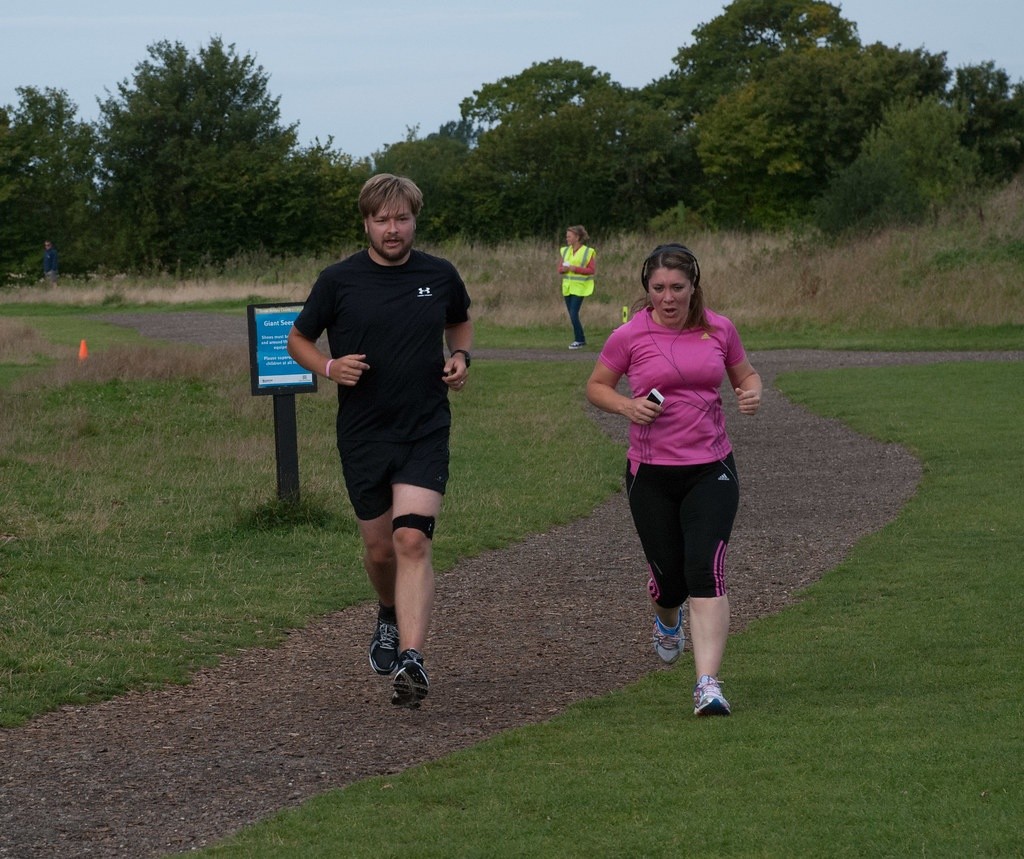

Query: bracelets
<box><xmin>325</xmin><ymin>359</ymin><xmax>334</xmax><ymax>377</ymax></box>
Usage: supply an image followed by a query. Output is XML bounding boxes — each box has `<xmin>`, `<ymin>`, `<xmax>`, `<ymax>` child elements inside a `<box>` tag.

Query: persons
<box><xmin>43</xmin><ymin>240</ymin><xmax>58</xmax><ymax>287</ymax></box>
<box><xmin>287</xmin><ymin>173</ymin><xmax>472</xmax><ymax>710</ymax></box>
<box><xmin>557</xmin><ymin>225</ymin><xmax>597</xmax><ymax>349</ymax></box>
<box><xmin>585</xmin><ymin>243</ymin><xmax>762</xmax><ymax>717</ymax></box>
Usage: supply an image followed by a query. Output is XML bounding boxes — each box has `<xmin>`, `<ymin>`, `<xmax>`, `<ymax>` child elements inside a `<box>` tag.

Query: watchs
<box><xmin>451</xmin><ymin>350</ymin><xmax>471</xmax><ymax>368</ymax></box>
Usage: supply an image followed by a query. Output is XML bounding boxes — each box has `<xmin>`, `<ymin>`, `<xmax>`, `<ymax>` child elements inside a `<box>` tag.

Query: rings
<box><xmin>461</xmin><ymin>381</ymin><xmax>464</xmax><ymax>385</ymax></box>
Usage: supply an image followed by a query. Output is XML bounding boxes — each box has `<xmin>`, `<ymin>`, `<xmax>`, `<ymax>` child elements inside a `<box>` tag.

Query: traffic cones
<box><xmin>79</xmin><ymin>338</ymin><xmax>89</xmax><ymax>361</ymax></box>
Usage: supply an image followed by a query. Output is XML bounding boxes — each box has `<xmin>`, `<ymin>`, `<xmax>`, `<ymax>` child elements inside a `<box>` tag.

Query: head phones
<box><xmin>642</xmin><ymin>248</ymin><xmax>701</xmax><ymax>294</ymax></box>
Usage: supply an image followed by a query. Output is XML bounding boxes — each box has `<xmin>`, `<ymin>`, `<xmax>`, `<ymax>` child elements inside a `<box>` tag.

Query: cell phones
<box><xmin>646</xmin><ymin>387</ymin><xmax>665</xmax><ymax>407</ymax></box>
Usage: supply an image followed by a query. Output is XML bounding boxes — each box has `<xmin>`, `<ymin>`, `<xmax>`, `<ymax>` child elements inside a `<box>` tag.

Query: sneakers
<box><xmin>646</xmin><ymin>577</ymin><xmax>685</xmax><ymax>664</ymax></box>
<box><xmin>691</xmin><ymin>674</ymin><xmax>732</xmax><ymax>715</ymax></box>
<box><xmin>367</xmin><ymin>608</ymin><xmax>399</xmax><ymax>676</ymax></box>
<box><xmin>568</xmin><ymin>341</ymin><xmax>587</xmax><ymax>350</ymax></box>
<box><xmin>391</xmin><ymin>658</ymin><xmax>430</xmax><ymax>711</ymax></box>
